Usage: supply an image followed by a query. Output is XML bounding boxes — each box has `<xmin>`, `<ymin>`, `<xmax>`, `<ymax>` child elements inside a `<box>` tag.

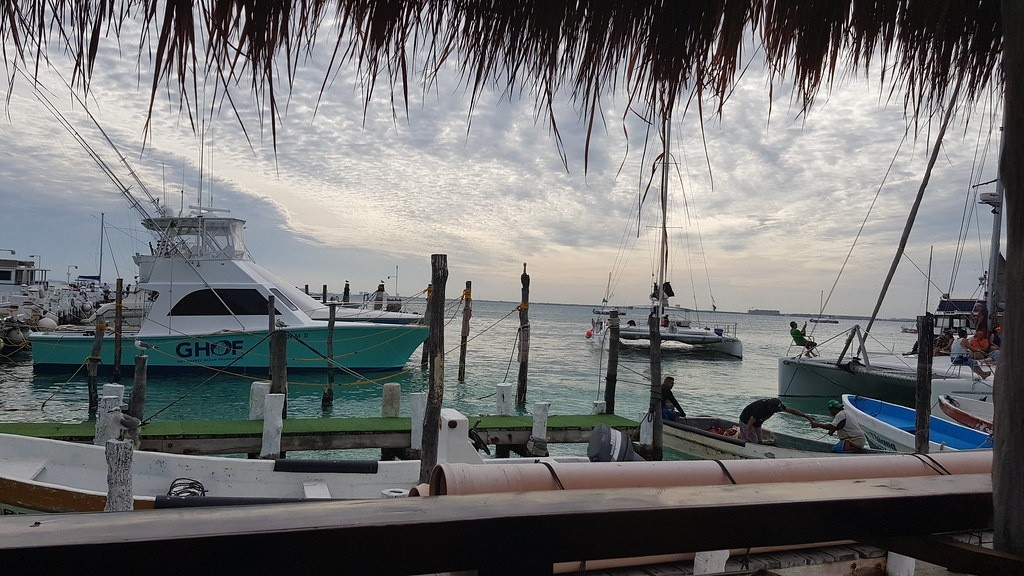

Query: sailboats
<box><xmin>809</xmin><ymin>290</ymin><xmax>839</xmax><ymax>324</ymax></box>
<box><xmin>585</xmin><ymin>69</ymin><xmax>741</xmax><ymax>359</ymax></box>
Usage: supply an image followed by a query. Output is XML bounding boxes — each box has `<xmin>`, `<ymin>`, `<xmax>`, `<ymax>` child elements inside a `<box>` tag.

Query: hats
<box><xmin>826</xmin><ymin>400</ymin><xmax>844</xmax><ymax>409</ymax></box>
<box><xmin>768</xmin><ymin>398</ymin><xmax>786</xmax><ymax>412</ymax></box>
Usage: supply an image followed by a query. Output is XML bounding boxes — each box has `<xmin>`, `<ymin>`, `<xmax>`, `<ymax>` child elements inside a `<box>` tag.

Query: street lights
<box><xmin>67</xmin><ymin>265</ymin><xmax>78</xmax><ymax>286</ymax></box>
<box><xmin>28</xmin><ymin>254</ymin><xmax>41</xmax><ymax>286</ymax></box>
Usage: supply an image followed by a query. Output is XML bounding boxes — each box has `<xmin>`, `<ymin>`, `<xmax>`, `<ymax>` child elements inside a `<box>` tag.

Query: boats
<box><xmin>936</xmin><ymin>391</ymin><xmax>994</xmax><ymax>436</ymax></box>
<box><xmin>0</xmin><ymin>422</ymin><xmax>649</xmax><ymax>517</ymax></box>
<box><xmin>661</xmin><ymin>412</ymin><xmax>879</xmax><ymax>460</ymax></box>
<box><xmin>10</xmin><ymin>44</ymin><xmax>433</xmax><ymax>375</ymax></box>
<box><xmin>841</xmin><ymin>392</ymin><xmax>993</xmax><ymax>454</ymax></box>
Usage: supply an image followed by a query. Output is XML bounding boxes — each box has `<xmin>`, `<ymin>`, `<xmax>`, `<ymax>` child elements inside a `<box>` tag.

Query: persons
<box><xmin>912</xmin><ymin>302</ymin><xmax>1001</xmax><ymax>379</ymax></box>
<box><xmin>810</xmin><ymin>400</ymin><xmax>865</xmax><ymax>453</ymax></box>
<box><xmin>739</xmin><ymin>398</ymin><xmax>816</xmax><ymax>444</ymax></box>
<box><xmin>790</xmin><ymin>321</ymin><xmax>818</xmax><ymax>358</ymax></box>
<box><xmin>661</xmin><ymin>377</ymin><xmax>686</xmax><ymax>422</ymax></box>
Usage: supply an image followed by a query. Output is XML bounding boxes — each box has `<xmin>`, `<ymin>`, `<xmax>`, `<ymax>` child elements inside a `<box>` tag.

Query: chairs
<box><xmin>943</xmin><ymin>352</ymin><xmax>974</xmax><ymax>381</ymax></box>
<box><xmin>786</xmin><ymin>336</ymin><xmax>820</xmax><ymax>357</ymax></box>
